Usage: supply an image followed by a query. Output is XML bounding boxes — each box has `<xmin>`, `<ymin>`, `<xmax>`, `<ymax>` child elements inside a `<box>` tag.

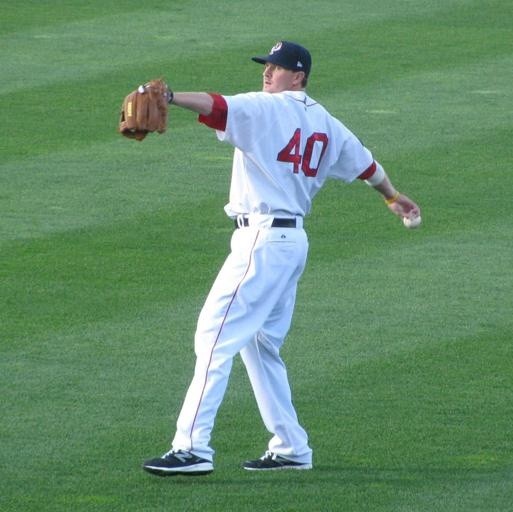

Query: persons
<box><xmin>142</xmin><ymin>39</ymin><xmax>420</xmax><ymax>475</ymax></box>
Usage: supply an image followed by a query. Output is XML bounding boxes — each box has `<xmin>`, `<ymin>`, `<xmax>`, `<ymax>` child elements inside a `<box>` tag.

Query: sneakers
<box><xmin>143</xmin><ymin>449</ymin><xmax>214</xmax><ymax>475</ymax></box>
<box><xmin>241</xmin><ymin>450</ymin><xmax>312</xmax><ymax>470</ymax></box>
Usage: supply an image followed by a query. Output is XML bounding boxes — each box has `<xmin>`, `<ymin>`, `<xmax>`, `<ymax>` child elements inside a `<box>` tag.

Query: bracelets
<box><xmin>385</xmin><ymin>191</ymin><xmax>400</xmax><ymax>205</ymax></box>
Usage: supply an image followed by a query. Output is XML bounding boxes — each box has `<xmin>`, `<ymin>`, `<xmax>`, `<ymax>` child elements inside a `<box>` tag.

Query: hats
<box><xmin>251</xmin><ymin>42</ymin><xmax>311</xmax><ymax>73</ymax></box>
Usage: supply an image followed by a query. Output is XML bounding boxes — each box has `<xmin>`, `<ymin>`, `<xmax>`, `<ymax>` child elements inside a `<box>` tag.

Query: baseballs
<box><xmin>404</xmin><ymin>216</ymin><xmax>421</xmax><ymax>229</ymax></box>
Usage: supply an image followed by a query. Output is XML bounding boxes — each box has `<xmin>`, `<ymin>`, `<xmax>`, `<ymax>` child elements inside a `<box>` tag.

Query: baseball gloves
<box><xmin>119</xmin><ymin>77</ymin><xmax>173</xmax><ymax>141</ymax></box>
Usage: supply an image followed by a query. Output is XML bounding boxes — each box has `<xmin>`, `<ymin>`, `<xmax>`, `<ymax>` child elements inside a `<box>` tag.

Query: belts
<box><xmin>233</xmin><ymin>217</ymin><xmax>296</xmax><ymax>228</ymax></box>
